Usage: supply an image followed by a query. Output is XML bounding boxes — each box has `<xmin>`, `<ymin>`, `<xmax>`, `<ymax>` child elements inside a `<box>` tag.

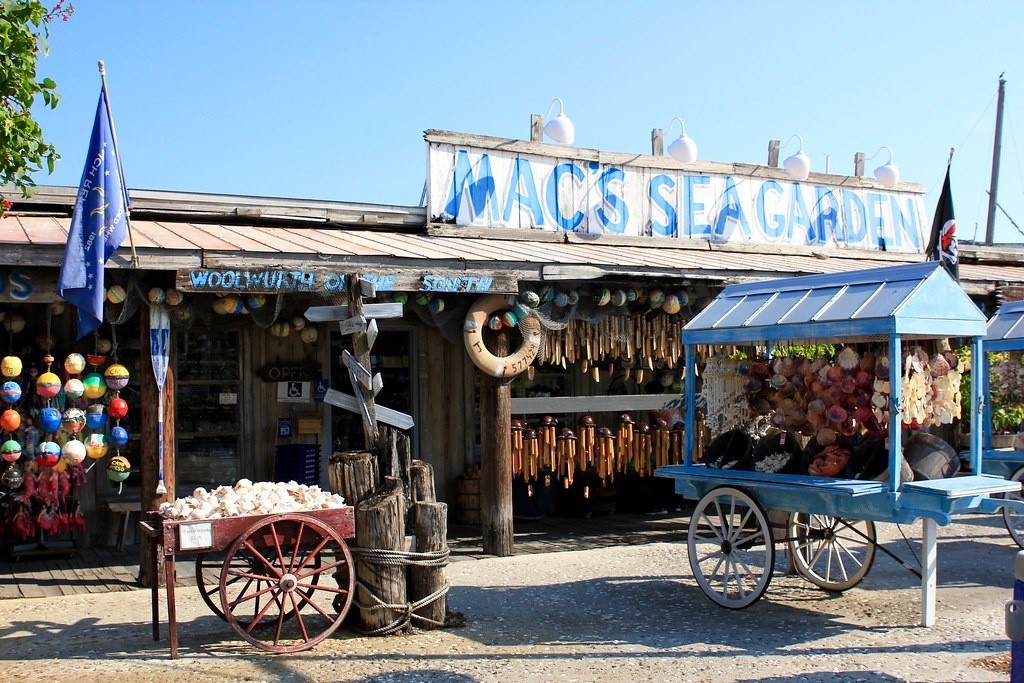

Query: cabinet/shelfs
<box><xmin>95</xmin><ymin>326</ymin><xmax>245</xmax><ymax>505</ymax></box>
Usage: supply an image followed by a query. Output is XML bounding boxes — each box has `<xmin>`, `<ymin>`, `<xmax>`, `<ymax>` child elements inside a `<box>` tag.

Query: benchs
<box><xmin>108</xmin><ymin>503</ymin><xmax>141</xmax><ymax>552</ymax></box>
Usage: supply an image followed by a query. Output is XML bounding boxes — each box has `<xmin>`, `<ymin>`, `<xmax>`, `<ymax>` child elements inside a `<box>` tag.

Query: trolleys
<box><xmin>139</xmin><ymin>503</ymin><xmax>359</xmax><ymax>664</ymax></box>
<box><xmin>651</xmin><ymin>260</ymin><xmax>1020</xmax><ymax>631</ymax></box>
<box><xmin>960</xmin><ymin>300</ymin><xmax>1024</xmax><ymax>550</ymax></box>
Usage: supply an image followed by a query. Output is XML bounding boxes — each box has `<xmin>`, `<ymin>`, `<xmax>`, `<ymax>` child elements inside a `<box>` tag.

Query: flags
<box><xmin>925</xmin><ymin>166</ymin><xmax>959</xmax><ymax>280</ymax></box>
<box><xmin>56</xmin><ymin>86</ymin><xmax>130</xmax><ymax>341</ymax></box>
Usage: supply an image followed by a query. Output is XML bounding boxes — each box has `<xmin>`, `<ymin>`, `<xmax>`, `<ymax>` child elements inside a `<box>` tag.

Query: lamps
<box><xmin>541</xmin><ymin>98</ymin><xmax>575</xmax><ymax>144</ymax></box>
<box><xmin>866</xmin><ymin>146</ymin><xmax>898</xmax><ymax>188</ymax></box>
<box><xmin>664</xmin><ymin>117</ymin><xmax>697</xmax><ymax>162</ymax></box>
<box><xmin>775</xmin><ymin>134</ymin><xmax>810</xmax><ymax>180</ymax></box>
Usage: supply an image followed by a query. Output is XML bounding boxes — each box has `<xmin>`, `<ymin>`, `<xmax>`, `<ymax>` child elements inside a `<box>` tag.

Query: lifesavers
<box><xmin>463</xmin><ymin>293</ymin><xmax>541</xmax><ymax>378</ymax></box>
